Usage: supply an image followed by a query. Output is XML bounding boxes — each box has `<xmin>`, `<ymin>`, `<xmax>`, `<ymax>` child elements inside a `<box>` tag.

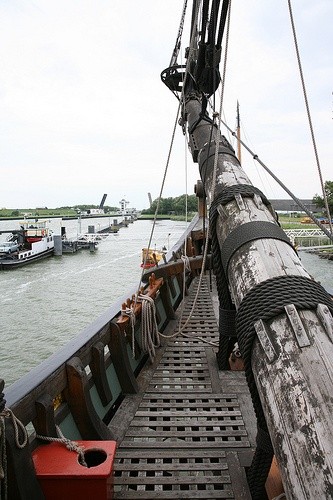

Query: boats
<box><xmin>0</xmin><ymin>220</ymin><xmax>55</xmax><ymax>268</ymax></box>
